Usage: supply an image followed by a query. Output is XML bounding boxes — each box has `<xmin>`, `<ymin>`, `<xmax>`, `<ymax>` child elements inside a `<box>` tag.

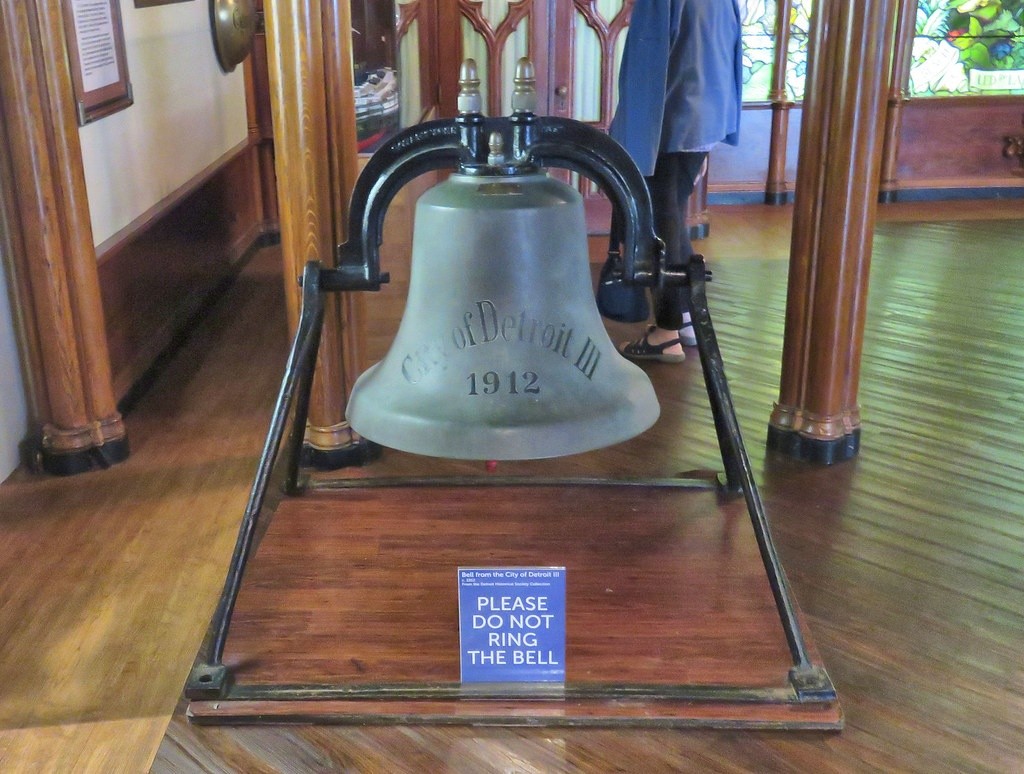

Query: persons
<box><xmin>610</xmin><ymin>0</ymin><xmax>743</xmax><ymax>362</ymax></box>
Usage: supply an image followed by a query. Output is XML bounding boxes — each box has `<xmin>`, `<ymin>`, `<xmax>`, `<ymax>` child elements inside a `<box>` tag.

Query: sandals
<box><xmin>619</xmin><ymin>335</ymin><xmax>685</xmax><ymax>363</ymax></box>
<box><xmin>645</xmin><ymin>321</ymin><xmax>698</xmax><ymax>346</ymax></box>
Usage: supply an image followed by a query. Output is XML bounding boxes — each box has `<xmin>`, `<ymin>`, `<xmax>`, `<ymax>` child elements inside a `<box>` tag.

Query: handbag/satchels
<box><xmin>596</xmin><ymin>211</ymin><xmax>650</xmax><ymax>323</ymax></box>
